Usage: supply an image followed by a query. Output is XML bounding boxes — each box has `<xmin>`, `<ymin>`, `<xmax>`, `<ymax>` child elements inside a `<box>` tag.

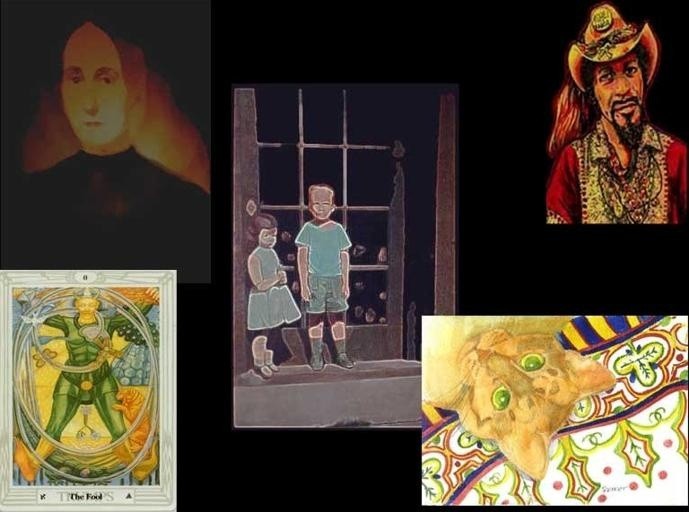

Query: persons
<box><xmin>0</xmin><ymin>9</ymin><xmax>211</xmax><ymax>279</ymax></box>
<box><xmin>14</xmin><ymin>286</ymin><xmax>160</xmax><ymax>483</ymax></box>
<box><xmin>545</xmin><ymin>2</ymin><xmax>687</xmax><ymax>225</ymax></box>
<box><xmin>244</xmin><ymin>216</ymin><xmax>301</xmax><ymax>379</ymax></box>
<box><xmin>293</xmin><ymin>183</ymin><xmax>355</xmax><ymax>372</ymax></box>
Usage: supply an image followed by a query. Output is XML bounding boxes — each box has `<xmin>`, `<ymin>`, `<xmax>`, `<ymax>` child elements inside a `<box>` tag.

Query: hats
<box><xmin>568</xmin><ymin>5</ymin><xmax>660</xmax><ymax>92</ymax></box>
